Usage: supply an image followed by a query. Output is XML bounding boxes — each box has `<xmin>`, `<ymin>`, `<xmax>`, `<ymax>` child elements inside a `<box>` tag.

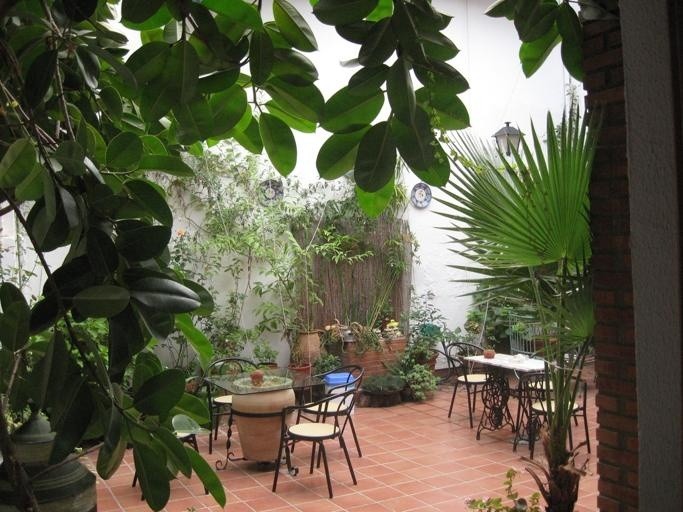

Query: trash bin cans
<box><xmin>323</xmin><ymin>373</ymin><xmax>355</xmax><ymax>415</ymax></box>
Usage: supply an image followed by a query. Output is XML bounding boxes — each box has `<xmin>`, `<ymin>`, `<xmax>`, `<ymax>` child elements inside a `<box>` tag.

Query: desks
<box><xmin>463</xmin><ymin>352</ymin><xmax>550</xmax><ymax>453</ymax></box>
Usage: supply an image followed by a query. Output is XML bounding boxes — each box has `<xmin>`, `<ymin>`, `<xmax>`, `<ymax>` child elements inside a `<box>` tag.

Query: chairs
<box><xmin>204</xmin><ymin>356</ymin><xmax>364</xmax><ymax>500</ymax></box>
<box><xmin>444</xmin><ymin>341</ymin><xmax>499</xmax><ymax>427</ymax></box>
<box><xmin>519</xmin><ymin>372</ymin><xmax>592</xmax><ymax>461</ymax></box>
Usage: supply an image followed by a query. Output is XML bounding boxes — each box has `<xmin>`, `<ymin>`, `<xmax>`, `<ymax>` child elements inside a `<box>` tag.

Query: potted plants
<box><xmin>359</xmin><ymin>306</ymin><xmax>443</xmax><ymax>408</ymax></box>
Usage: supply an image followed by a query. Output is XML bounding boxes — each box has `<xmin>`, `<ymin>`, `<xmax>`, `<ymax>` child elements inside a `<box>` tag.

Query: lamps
<box><xmin>492</xmin><ymin>121</ymin><xmax>525</xmax><ymax>158</ymax></box>
